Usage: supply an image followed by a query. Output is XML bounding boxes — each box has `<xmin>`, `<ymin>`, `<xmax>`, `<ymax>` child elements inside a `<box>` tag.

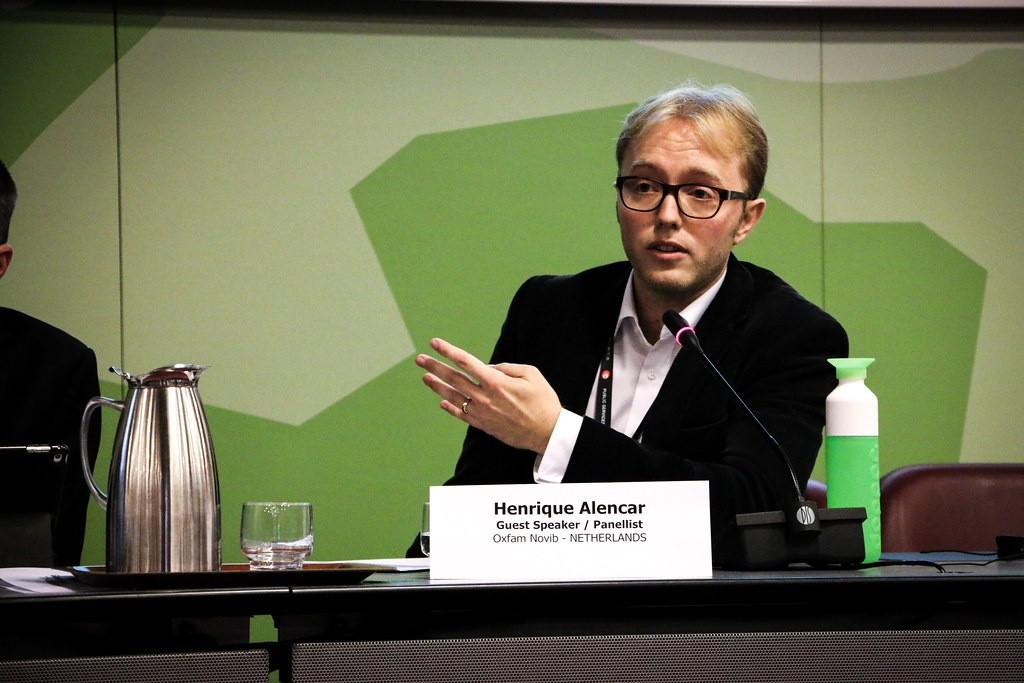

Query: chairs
<box><xmin>880</xmin><ymin>463</ymin><xmax>1024</xmax><ymax>554</ymax></box>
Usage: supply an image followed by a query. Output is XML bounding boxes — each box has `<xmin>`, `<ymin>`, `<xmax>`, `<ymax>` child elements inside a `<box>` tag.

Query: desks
<box><xmin>0</xmin><ymin>551</ymin><xmax>1024</xmax><ymax>683</ymax></box>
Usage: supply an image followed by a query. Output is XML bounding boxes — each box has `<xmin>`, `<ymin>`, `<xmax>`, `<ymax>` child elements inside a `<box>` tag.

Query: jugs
<box><xmin>77</xmin><ymin>363</ymin><xmax>222</xmax><ymax>572</ymax></box>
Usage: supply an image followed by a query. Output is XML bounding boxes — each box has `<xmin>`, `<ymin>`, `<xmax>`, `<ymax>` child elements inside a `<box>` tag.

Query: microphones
<box><xmin>662</xmin><ymin>310</ymin><xmax>868</xmax><ymax>572</ymax></box>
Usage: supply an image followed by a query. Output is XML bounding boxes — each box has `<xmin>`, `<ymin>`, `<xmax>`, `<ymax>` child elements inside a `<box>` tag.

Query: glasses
<box><xmin>615</xmin><ymin>175</ymin><xmax>752</xmax><ymax>219</ymax></box>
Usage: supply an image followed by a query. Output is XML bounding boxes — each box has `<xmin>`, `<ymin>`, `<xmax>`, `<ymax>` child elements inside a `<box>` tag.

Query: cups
<box><xmin>239</xmin><ymin>502</ymin><xmax>314</xmax><ymax>570</ymax></box>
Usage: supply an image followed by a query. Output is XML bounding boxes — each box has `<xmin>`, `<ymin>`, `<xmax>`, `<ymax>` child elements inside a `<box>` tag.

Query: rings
<box><xmin>459</xmin><ymin>397</ymin><xmax>473</xmax><ymax>415</ymax></box>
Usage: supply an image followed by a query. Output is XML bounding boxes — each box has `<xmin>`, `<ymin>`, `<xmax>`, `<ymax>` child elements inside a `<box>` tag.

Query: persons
<box><xmin>402</xmin><ymin>84</ymin><xmax>848</xmax><ymax>558</ymax></box>
<box><xmin>0</xmin><ymin>159</ymin><xmax>102</xmax><ymax>569</ymax></box>
